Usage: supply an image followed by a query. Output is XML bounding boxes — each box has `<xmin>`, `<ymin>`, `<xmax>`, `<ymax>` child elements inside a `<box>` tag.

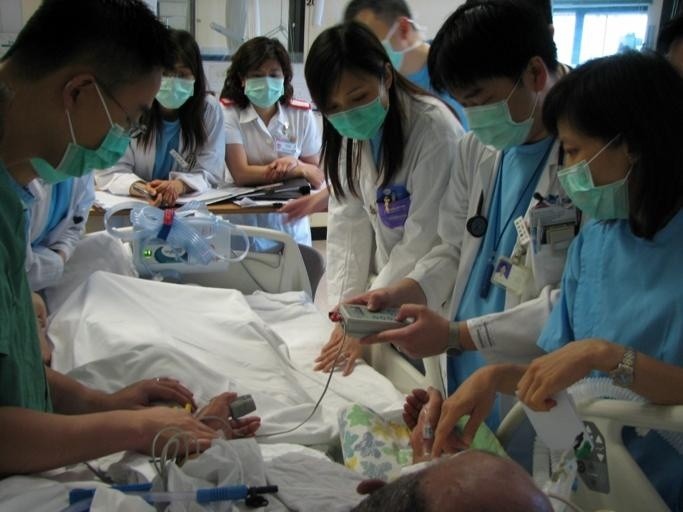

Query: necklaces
<box><xmin>476</xmin><ymin>135</ymin><xmax>557</xmax><ymax>299</ymax></box>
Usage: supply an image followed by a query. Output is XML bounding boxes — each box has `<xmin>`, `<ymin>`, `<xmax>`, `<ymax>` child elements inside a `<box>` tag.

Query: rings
<box><xmin>340</xmin><ymin>353</ymin><xmax>346</xmax><ymax>359</ymax></box>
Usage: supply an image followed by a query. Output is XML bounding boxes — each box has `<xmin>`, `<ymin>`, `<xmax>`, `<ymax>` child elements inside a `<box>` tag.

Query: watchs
<box><xmin>606</xmin><ymin>347</ymin><xmax>634</xmax><ymax>387</ymax></box>
<box><xmin>445</xmin><ymin>321</ymin><xmax>464</xmax><ymax>358</ymax></box>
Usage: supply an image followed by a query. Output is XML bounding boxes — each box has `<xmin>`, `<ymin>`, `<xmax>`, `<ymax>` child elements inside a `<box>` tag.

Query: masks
<box><xmin>32</xmin><ymin>78</ymin><xmax>132</xmax><ymax>185</ymax></box>
<box><xmin>324</xmin><ymin>78</ymin><xmax>390</xmax><ymax>141</ymax></box>
<box><xmin>463</xmin><ymin>77</ymin><xmax>538</xmax><ymax>152</ymax></box>
<box><xmin>380</xmin><ymin>20</ymin><xmax>427</xmax><ymax>70</ymax></box>
<box><xmin>556</xmin><ymin>136</ymin><xmax>631</xmax><ymax>220</ymax></box>
<box><xmin>154</xmin><ymin>78</ymin><xmax>196</xmax><ymax>110</ymax></box>
<box><xmin>243</xmin><ymin>76</ymin><xmax>285</xmax><ymax>109</ymax></box>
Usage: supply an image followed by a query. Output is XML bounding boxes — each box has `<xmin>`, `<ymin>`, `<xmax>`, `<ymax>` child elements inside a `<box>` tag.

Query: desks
<box><xmin>82</xmin><ymin>197</ymin><xmax>285</xmax><ymax>217</ymax></box>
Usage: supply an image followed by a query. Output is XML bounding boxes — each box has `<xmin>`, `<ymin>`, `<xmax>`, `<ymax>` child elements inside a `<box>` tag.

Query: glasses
<box><xmin>99</xmin><ymin>83</ymin><xmax>147</xmax><ymax>138</ymax></box>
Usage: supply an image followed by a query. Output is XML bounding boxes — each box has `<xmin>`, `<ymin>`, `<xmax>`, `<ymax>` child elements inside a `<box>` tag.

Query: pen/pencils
<box><xmin>133</xmin><ymin>186</ymin><xmax>156</xmax><ymax>200</ymax></box>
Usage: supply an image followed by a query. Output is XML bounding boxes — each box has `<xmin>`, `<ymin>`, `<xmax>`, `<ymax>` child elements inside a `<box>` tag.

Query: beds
<box><xmin>35</xmin><ymin>225</ymin><xmax>683</xmax><ymax>511</ymax></box>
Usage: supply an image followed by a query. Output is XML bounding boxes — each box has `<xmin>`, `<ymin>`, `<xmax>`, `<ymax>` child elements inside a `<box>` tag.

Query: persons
<box><xmin>181</xmin><ymin>385</ymin><xmax>555</xmax><ymax>512</ymax></box>
<box><xmin>218</xmin><ymin>36</ymin><xmax>323</xmax><ymax>189</ymax></box>
<box><xmin>656</xmin><ymin>11</ymin><xmax>682</xmax><ymax>72</ymax></box>
<box><xmin>92</xmin><ymin>28</ymin><xmax>225</xmax><ymax>209</ymax></box>
<box><xmin>465</xmin><ymin>1</ymin><xmax>555</xmax><ymax>42</ymax></box>
<box><xmin>343</xmin><ymin>1</ymin><xmax>468</xmax><ymax>132</ymax></box>
<box><xmin>0</xmin><ymin>0</ymin><xmax>260</xmax><ymax>479</ymax></box>
<box><xmin>303</xmin><ymin>21</ymin><xmax>467</xmax><ymax>373</ymax></box>
<box><xmin>21</xmin><ymin>166</ymin><xmax>95</xmax><ymax>291</ymax></box>
<box><xmin>327</xmin><ymin>3</ymin><xmax>593</xmax><ymax>359</ymax></box>
<box><xmin>428</xmin><ymin>52</ymin><xmax>682</xmax><ymax>458</ymax></box>
